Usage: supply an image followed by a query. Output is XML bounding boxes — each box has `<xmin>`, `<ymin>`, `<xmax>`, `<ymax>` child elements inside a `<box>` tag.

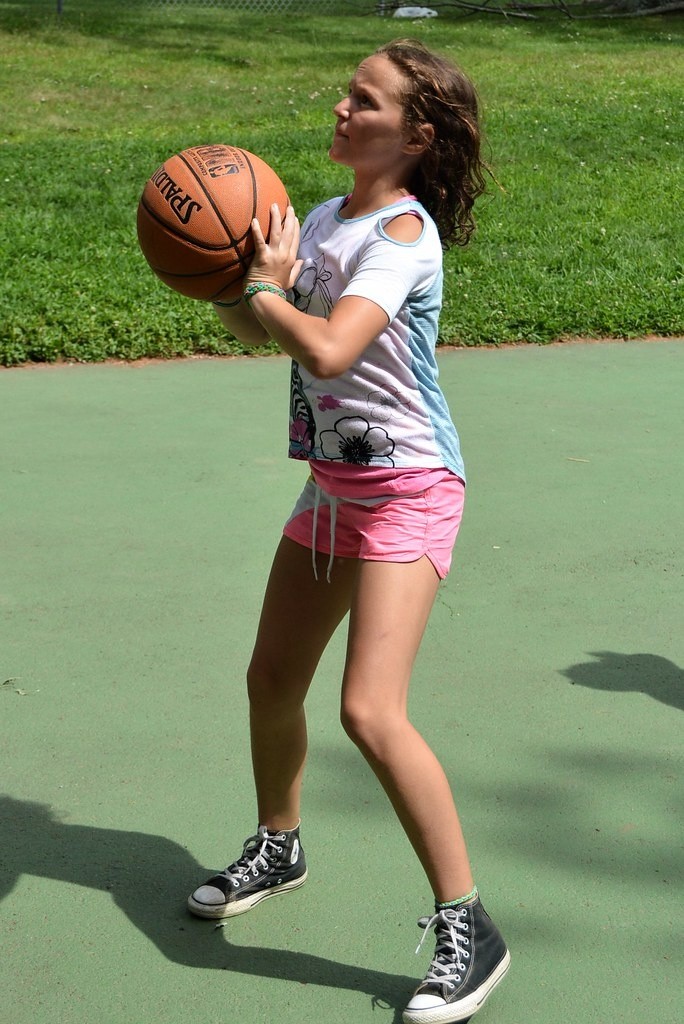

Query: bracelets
<box><xmin>210</xmin><ymin>280</ymin><xmax>288</xmax><ymax>308</ymax></box>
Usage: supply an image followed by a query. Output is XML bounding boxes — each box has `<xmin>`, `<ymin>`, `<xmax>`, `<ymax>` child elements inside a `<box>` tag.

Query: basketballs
<box><xmin>136</xmin><ymin>143</ymin><xmax>294</xmax><ymax>302</ymax></box>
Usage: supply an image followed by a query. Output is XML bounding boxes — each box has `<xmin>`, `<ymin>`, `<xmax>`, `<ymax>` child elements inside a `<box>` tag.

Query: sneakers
<box><xmin>188</xmin><ymin>819</ymin><xmax>307</xmax><ymax>919</ymax></box>
<box><xmin>402</xmin><ymin>891</ymin><xmax>512</xmax><ymax>1024</ymax></box>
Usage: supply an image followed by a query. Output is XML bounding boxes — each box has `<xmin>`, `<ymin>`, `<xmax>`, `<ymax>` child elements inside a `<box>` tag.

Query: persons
<box><xmin>184</xmin><ymin>43</ymin><xmax>512</xmax><ymax>1024</ymax></box>
